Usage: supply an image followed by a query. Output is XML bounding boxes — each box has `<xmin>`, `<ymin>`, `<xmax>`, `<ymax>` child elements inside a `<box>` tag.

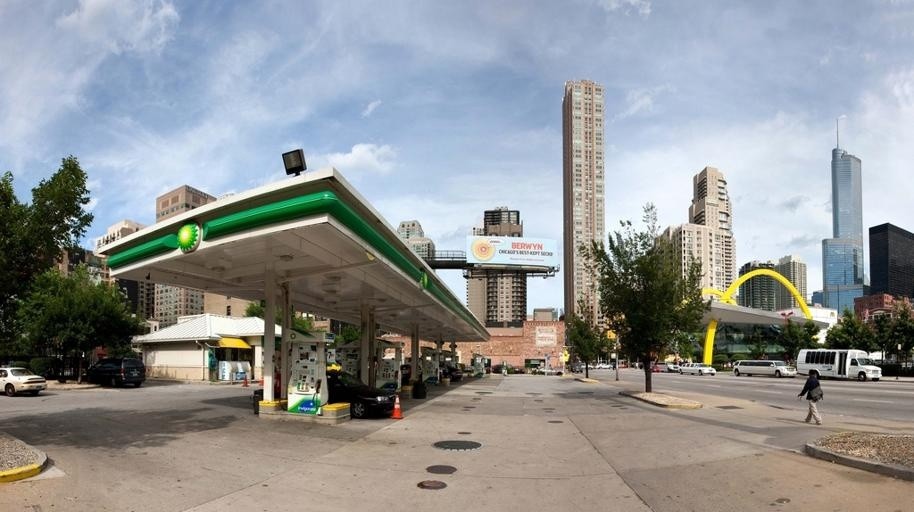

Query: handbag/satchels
<box><xmin>810</xmin><ymin>386</ymin><xmax>824</xmax><ymax>402</ymax></box>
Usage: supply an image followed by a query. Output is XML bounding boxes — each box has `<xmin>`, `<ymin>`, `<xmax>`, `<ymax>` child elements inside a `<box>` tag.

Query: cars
<box><xmin>0</xmin><ymin>367</ymin><xmax>49</xmax><ymax>397</ymax></box>
<box><xmin>399</xmin><ymin>364</ymin><xmax>422</xmax><ymax>387</ymax></box>
<box><xmin>440</xmin><ymin>362</ymin><xmax>563</xmax><ymax>382</ymax></box>
<box><xmin>569</xmin><ymin>358</ymin><xmax>680</xmax><ymax>375</ymax></box>
<box><xmin>324</xmin><ymin>370</ymin><xmax>394</xmax><ymax>419</ymax></box>
<box><xmin>678</xmin><ymin>363</ymin><xmax>716</xmax><ymax>376</ymax></box>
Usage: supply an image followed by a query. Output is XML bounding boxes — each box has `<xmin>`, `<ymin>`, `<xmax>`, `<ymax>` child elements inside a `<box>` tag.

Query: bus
<box><xmin>796</xmin><ymin>347</ymin><xmax>882</xmax><ymax>382</ymax></box>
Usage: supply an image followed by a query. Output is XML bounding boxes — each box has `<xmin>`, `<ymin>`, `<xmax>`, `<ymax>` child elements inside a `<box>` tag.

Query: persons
<box><xmin>798</xmin><ymin>368</ymin><xmax>824</xmax><ymax>425</ymax></box>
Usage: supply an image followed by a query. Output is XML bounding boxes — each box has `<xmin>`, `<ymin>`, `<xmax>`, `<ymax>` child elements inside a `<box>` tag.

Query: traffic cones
<box><xmin>258</xmin><ymin>376</ymin><xmax>264</xmax><ymax>386</ymax></box>
<box><xmin>389</xmin><ymin>394</ymin><xmax>404</xmax><ymax>420</ymax></box>
<box><xmin>240</xmin><ymin>375</ymin><xmax>249</xmax><ymax>387</ymax></box>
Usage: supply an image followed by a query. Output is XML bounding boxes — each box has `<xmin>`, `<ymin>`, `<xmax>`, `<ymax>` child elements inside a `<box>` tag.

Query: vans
<box><xmin>85</xmin><ymin>356</ymin><xmax>145</xmax><ymax>388</ymax></box>
<box><xmin>733</xmin><ymin>359</ymin><xmax>797</xmax><ymax>377</ymax></box>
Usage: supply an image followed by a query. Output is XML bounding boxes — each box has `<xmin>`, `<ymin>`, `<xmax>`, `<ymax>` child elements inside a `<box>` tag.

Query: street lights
<box><xmin>835</xmin><ymin>114</ymin><xmax>846</xmax><ymax>148</ymax></box>
<box><xmin>780</xmin><ymin>312</ymin><xmax>792</xmax><ymax>327</ymax></box>
